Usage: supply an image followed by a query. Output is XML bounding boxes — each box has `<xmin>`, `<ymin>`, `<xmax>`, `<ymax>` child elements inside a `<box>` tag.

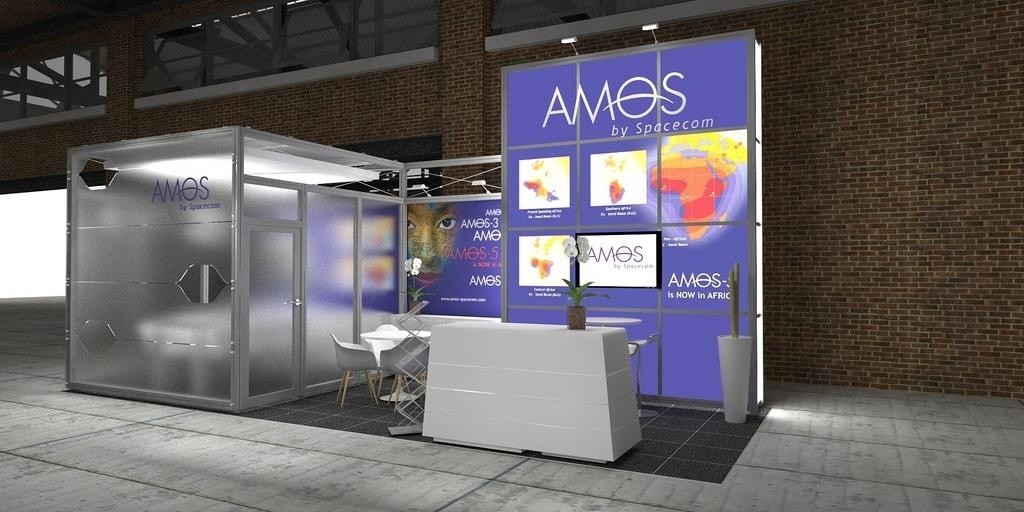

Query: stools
<box><xmin>629</xmin><ymin>333</ymin><xmax>663</xmax><ymax>416</ymax></box>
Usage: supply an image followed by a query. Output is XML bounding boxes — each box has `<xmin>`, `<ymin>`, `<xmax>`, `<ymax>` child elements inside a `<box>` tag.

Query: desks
<box><xmin>586</xmin><ymin>315</ymin><xmax>643</xmax><ymax>329</ymax></box>
<box><xmin>391</xmin><ymin>315</ymin><xmax>501</xmax><ymax>331</ymax></box>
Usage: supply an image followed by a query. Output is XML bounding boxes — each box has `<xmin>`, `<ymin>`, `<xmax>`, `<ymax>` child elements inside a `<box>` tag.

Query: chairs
<box><xmin>331</xmin><ymin>322</ymin><xmax>431</xmax><ymax>408</ymax></box>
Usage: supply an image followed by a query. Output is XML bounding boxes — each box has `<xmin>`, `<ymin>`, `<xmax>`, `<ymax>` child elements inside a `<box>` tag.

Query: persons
<box><xmin>406</xmin><ymin>201</ymin><xmax>460</xmax><ymax>296</ymax></box>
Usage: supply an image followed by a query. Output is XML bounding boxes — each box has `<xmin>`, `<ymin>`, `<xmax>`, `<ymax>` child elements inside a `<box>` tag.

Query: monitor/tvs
<box><xmin>576</xmin><ymin>232</ymin><xmax>662</xmax><ymax>290</ymax></box>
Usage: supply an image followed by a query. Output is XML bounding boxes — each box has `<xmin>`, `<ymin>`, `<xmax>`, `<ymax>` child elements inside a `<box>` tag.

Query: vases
<box><xmin>566</xmin><ymin>307</ymin><xmax>586</xmax><ymax>328</ymax></box>
<box><xmin>410</xmin><ymin>301</ymin><xmax>421</xmax><ymax>315</ymax></box>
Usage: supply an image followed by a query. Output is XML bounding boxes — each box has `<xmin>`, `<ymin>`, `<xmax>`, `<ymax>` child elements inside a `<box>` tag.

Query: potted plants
<box><xmin>718</xmin><ymin>259</ymin><xmax>752</xmax><ymax>424</ymax></box>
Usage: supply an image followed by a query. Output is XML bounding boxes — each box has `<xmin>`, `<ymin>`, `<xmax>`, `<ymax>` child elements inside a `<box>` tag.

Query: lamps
<box><xmin>641</xmin><ymin>23</ymin><xmax>659</xmax><ymax>45</ymax></box>
<box><xmin>412</xmin><ymin>184</ymin><xmax>432</xmax><ymax>196</ymax></box>
<box><xmin>561</xmin><ymin>36</ymin><xmax>579</xmax><ymax>56</ymax></box>
<box><xmin>471</xmin><ymin>179</ymin><xmax>490</xmax><ymax>193</ymax></box>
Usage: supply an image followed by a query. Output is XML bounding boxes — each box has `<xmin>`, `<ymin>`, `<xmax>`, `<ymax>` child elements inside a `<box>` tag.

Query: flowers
<box><xmin>557</xmin><ymin>236</ymin><xmax>611</xmax><ymax>306</ymax></box>
<box><xmin>402</xmin><ymin>257</ymin><xmax>436</xmax><ymax>302</ymax></box>
